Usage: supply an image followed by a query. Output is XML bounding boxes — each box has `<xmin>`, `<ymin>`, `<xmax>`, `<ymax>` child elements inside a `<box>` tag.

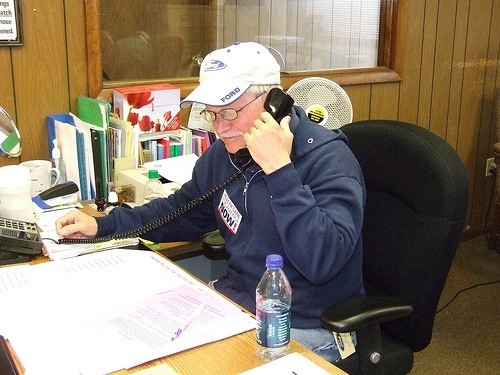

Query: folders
<box><xmin>77</xmin><ymin>96</ymin><xmax>110</xmax><ymax>206</ymax></box>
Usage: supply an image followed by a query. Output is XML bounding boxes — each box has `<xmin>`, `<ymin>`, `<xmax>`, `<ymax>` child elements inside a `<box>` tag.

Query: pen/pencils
<box><xmin>171</xmin><ymin>303</ymin><xmax>207</xmax><ymax>342</ymax></box>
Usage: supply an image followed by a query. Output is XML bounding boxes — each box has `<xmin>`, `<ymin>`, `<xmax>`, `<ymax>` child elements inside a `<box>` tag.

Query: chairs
<box><xmin>321</xmin><ymin>120</ymin><xmax>466</xmax><ymax>374</ymax></box>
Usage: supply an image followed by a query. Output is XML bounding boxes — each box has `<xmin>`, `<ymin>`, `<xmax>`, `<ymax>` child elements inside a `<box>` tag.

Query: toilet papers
<box><xmin>0</xmin><ymin>164</ymin><xmax>34</xmax><ymax>224</ymax></box>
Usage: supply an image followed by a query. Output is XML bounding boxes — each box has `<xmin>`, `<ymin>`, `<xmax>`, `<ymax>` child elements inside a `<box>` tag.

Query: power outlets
<box><xmin>485</xmin><ymin>157</ymin><xmax>496</xmax><ymax>177</ymax></box>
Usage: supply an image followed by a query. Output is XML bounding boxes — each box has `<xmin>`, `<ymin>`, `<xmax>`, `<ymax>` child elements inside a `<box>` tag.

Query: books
<box><xmin>46</xmin><ymin>96</ymin><xmax>218</xmax><ymax>205</ymax></box>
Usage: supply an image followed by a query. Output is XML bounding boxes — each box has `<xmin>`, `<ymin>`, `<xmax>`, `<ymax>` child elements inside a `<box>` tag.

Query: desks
<box><xmin>0</xmin><ymin>210</ymin><xmax>350</xmax><ymax>375</ymax></box>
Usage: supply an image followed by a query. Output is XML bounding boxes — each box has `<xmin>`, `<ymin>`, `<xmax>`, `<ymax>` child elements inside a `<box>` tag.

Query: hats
<box><xmin>179</xmin><ymin>42</ymin><xmax>281</xmax><ymax>109</ymax></box>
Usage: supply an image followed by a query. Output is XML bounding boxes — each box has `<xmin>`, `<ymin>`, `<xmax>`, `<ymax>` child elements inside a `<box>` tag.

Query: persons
<box><xmin>55</xmin><ymin>40</ymin><xmax>367</xmax><ymax>365</ymax></box>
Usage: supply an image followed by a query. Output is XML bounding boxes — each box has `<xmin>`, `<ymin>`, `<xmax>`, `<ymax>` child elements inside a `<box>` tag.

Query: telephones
<box><xmin>234</xmin><ymin>88</ymin><xmax>294</xmax><ymax>168</ymax></box>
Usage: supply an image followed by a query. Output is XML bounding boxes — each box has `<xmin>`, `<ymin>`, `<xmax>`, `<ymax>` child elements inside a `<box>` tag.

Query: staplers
<box><xmin>32</xmin><ymin>181</ymin><xmax>84</xmax><ymax>213</ymax></box>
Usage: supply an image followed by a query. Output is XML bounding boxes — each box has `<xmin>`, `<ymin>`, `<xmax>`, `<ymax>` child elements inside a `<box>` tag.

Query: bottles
<box><xmin>255</xmin><ymin>254</ymin><xmax>292</xmax><ymax>365</ymax></box>
<box><xmin>142</xmin><ymin>170</ymin><xmax>164</xmax><ymax>203</ymax></box>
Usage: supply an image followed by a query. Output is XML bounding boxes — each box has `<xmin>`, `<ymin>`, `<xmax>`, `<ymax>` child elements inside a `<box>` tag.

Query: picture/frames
<box><xmin>0</xmin><ymin>0</ymin><xmax>24</xmax><ymax>47</ymax></box>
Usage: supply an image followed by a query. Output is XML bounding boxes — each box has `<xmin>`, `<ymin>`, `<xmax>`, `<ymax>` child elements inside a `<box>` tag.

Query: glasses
<box><xmin>199</xmin><ymin>90</ymin><xmax>268</xmax><ymax>122</ymax></box>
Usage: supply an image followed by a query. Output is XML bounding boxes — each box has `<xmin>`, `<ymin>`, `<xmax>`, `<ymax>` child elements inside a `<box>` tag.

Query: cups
<box><xmin>19</xmin><ymin>159</ymin><xmax>60</xmax><ymax>198</ymax></box>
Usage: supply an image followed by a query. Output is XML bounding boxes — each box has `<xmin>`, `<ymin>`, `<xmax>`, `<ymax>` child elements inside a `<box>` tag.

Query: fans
<box><xmin>285</xmin><ymin>77</ymin><xmax>354</xmax><ymax>130</ymax></box>
